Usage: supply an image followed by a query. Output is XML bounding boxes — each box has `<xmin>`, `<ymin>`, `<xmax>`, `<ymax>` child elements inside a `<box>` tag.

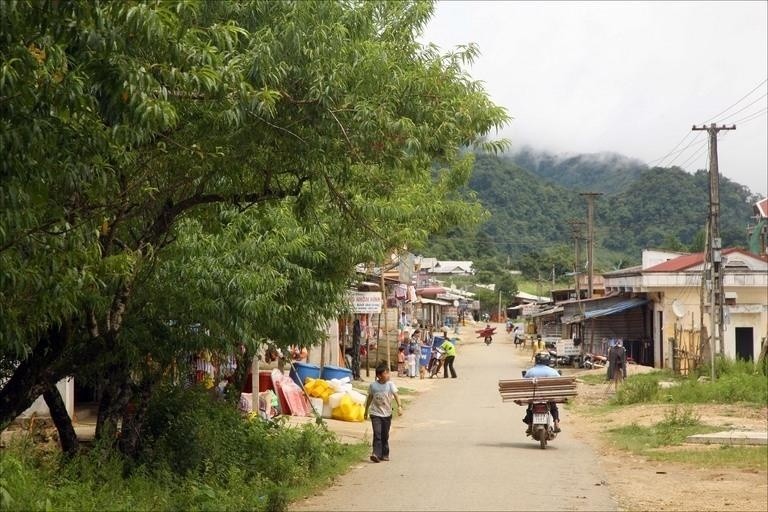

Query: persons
<box><xmin>485</xmin><ymin>324</ymin><xmax>491</xmax><ymax>339</ymax></box>
<box><xmin>406</xmin><ymin>348</ymin><xmax>417</xmax><ymax>377</ymax></box>
<box><xmin>522</xmin><ymin>351</ymin><xmax>563</xmax><ymax>433</ymax></box>
<box><xmin>406</xmin><ymin>330</ymin><xmax>433</xmax><ymax>376</ymax></box>
<box><xmin>439</xmin><ymin>338</ymin><xmax>456</xmax><ymax>378</ymax></box>
<box><xmin>531</xmin><ymin>335</ymin><xmax>545</xmax><ymax>361</ymax></box>
<box><xmin>362</xmin><ymin>367</ymin><xmax>403</xmax><ymax>462</ymax></box>
<box><xmin>397</xmin><ymin>346</ymin><xmax>405</xmax><ymax>377</ymax></box>
<box><xmin>514</xmin><ymin>326</ymin><xmax>522</xmax><ymax>348</ymax></box>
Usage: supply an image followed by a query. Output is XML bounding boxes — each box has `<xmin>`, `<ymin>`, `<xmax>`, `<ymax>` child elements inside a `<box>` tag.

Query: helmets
<box><xmin>534</xmin><ymin>352</ymin><xmax>550</xmax><ymax>365</ymax></box>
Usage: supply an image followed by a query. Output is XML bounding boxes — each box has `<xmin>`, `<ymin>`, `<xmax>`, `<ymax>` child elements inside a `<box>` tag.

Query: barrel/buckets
<box><xmin>289</xmin><ymin>362</ymin><xmax>352</xmax><ymax>391</ymax></box>
<box><xmin>401</xmin><ymin>336</ymin><xmax>449</xmax><ymax>366</ymax></box>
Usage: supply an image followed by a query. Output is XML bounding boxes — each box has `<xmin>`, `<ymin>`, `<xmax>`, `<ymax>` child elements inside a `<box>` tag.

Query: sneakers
<box><xmin>370</xmin><ymin>455</ymin><xmax>388</xmax><ymax>462</ymax></box>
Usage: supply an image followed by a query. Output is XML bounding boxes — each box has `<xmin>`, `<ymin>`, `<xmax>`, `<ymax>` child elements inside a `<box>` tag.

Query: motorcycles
<box><xmin>484</xmin><ymin>332</ymin><xmax>492</xmax><ymax>346</ymax></box>
<box><xmin>425</xmin><ymin>345</ymin><xmax>453</xmax><ymax>378</ymax></box>
<box><xmin>545</xmin><ymin>340</ymin><xmax>570</xmax><ymax>367</ymax></box>
<box><xmin>609</xmin><ymin>347</ymin><xmax>626</xmax><ymax>380</ymax></box>
<box><xmin>506</xmin><ymin>323</ymin><xmax>512</xmax><ymax>334</ymax></box>
<box><xmin>521</xmin><ymin>369</ymin><xmax>563</xmax><ymax>449</ymax></box>
